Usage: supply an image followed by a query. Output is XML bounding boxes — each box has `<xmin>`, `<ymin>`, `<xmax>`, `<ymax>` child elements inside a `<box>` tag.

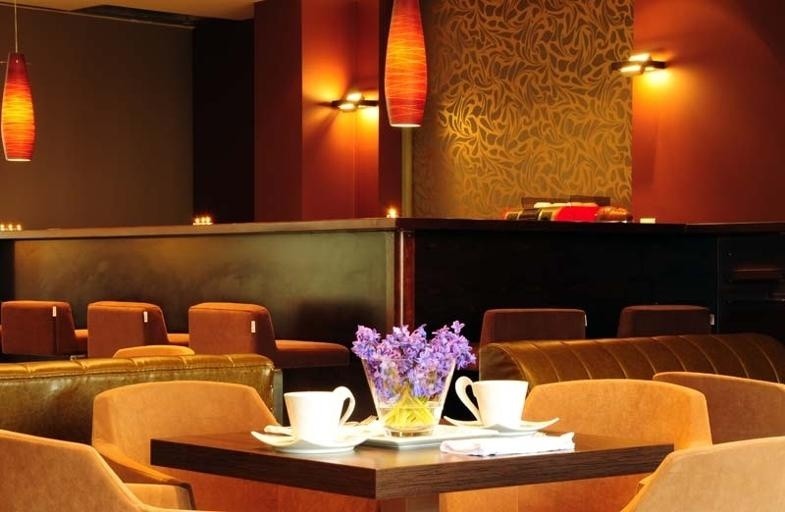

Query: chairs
<box><xmin>0</xmin><ymin>301</ymin><xmax>785</xmax><ymax>512</ymax></box>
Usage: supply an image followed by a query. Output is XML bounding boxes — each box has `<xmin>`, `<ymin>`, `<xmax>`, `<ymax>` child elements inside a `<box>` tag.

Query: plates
<box><xmin>358</xmin><ymin>424</ymin><xmax>499</xmax><ymax>450</ymax></box>
<box><xmin>249</xmin><ymin>421</ymin><xmax>386</xmax><ymax>456</ymax></box>
<box><xmin>442</xmin><ymin>413</ymin><xmax>561</xmax><ymax>433</ymax></box>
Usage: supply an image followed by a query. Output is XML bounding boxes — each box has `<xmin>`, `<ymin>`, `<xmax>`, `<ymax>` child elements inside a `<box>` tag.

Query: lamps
<box><xmin>384</xmin><ymin>0</ymin><xmax>429</xmax><ymax>128</ymax></box>
<box><xmin>1</xmin><ymin>0</ymin><xmax>37</xmax><ymax>163</ymax></box>
<box><xmin>612</xmin><ymin>51</ymin><xmax>666</xmax><ymax>76</ymax></box>
<box><xmin>331</xmin><ymin>90</ymin><xmax>378</xmax><ymax>113</ymax></box>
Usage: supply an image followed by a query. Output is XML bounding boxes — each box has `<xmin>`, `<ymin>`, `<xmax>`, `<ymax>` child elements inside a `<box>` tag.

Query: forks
<box><xmin>356</xmin><ymin>415</ymin><xmax>378</xmax><ymax>427</ymax></box>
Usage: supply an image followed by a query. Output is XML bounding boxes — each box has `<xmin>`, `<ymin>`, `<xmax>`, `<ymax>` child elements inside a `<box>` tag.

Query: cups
<box><xmin>281</xmin><ymin>385</ymin><xmax>357</xmax><ymax>442</ymax></box>
<box><xmin>453</xmin><ymin>375</ymin><xmax>529</xmax><ymax>426</ymax></box>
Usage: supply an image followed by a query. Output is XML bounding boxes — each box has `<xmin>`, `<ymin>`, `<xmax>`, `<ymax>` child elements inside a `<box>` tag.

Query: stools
<box><xmin>726</xmin><ymin>261</ymin><xmax>777</xmax><ymax>336</ymax></box>
